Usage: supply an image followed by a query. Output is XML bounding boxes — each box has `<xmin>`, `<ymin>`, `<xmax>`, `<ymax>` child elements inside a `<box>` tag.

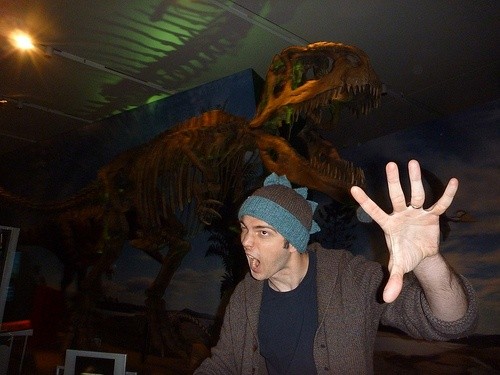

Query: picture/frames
<box><xmin>64</xmin><ymin>350</ymin><xmax>128</xmax><ymax>375</ymax></box>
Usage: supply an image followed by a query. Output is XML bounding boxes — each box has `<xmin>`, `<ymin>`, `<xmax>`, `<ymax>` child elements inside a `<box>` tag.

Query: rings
<box><xmin>411</xmin><ymin>205</ymin><xmax>423</xmax><ymax>209</ymax></box>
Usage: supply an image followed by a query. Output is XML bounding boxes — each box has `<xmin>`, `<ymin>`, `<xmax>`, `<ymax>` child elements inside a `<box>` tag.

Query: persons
<box><xmin>191</xmin><ymin>160</ymin><xmax>480</xmax><ymax>375</ymax></box>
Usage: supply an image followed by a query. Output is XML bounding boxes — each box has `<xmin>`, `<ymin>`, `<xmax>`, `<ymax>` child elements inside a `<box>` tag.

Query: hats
<box><xmin>238</xmin><ymin>172</ymin><xmax>322</xmax><ymax>254</ymax></box>
